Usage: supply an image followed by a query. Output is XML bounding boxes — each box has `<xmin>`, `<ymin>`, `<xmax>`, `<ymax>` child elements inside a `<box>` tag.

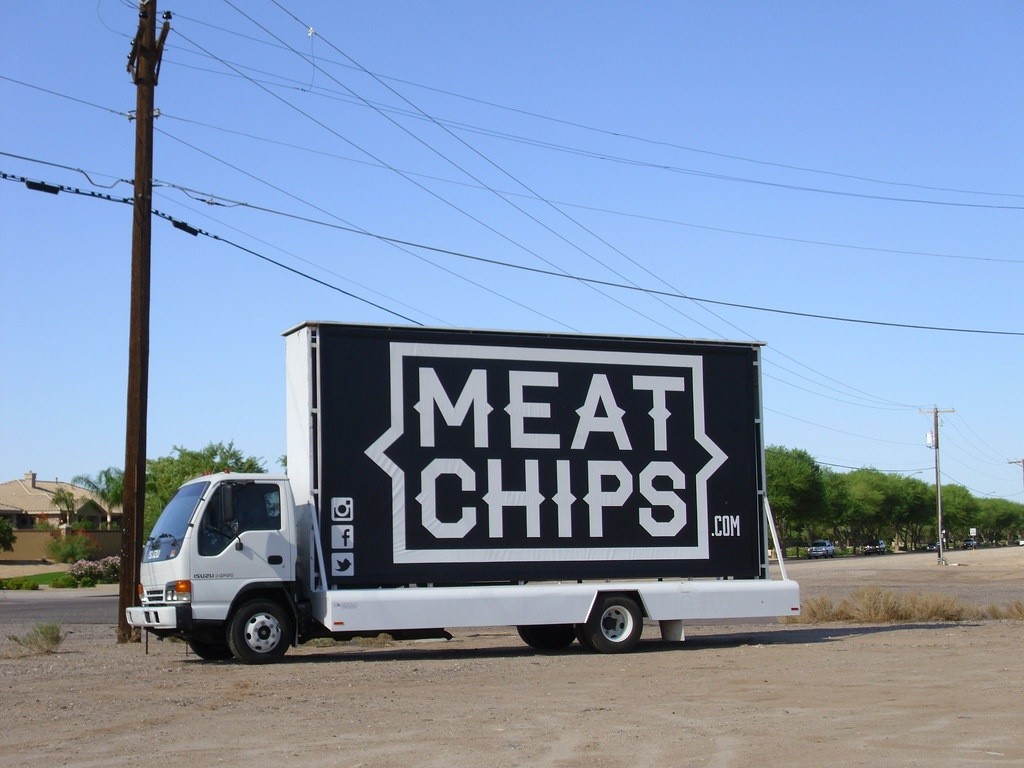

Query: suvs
<box><xmin>963</xmin><ymin>538</ymin><xmax>977</xmax><ymax>549</ymax></box>
<box><xmin>808</xmin><ymin>540</ymin><xmax>835</xmax><ymax>558</ymax></box>
<box><xmin>1019</xmin><ymin>541</ymin><xmax>1024</xmax><ymax>546</ymax></box>
<box><xmin>863</xmin><ymin>539</ymin><xmax>885</xmax><ymax>555</ymax></box>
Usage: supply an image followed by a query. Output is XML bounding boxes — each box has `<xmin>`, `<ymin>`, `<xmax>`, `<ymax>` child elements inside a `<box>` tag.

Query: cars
<box><xmin>926</xmin><ymin>544</ymin><xmax>938</xmax><ymax>551</ymax></box>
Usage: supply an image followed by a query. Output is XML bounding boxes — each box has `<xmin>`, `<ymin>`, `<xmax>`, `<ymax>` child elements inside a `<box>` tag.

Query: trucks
<box><xmin>125</xmin><ymin>318</ymin><xmax>800</xmax><ymax>664</ymax></box>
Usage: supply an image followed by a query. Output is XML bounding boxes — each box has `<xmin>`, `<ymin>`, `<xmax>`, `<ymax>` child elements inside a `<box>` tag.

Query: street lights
<box><xmin>979</xmin><ymin>491</ymin><xmax>996</xmax><ymax>499</ymax></box>
<box><xmin>906</xmin><ymin>472</ymin><xmax>923</xmax><ymax>478</ymax></box>
